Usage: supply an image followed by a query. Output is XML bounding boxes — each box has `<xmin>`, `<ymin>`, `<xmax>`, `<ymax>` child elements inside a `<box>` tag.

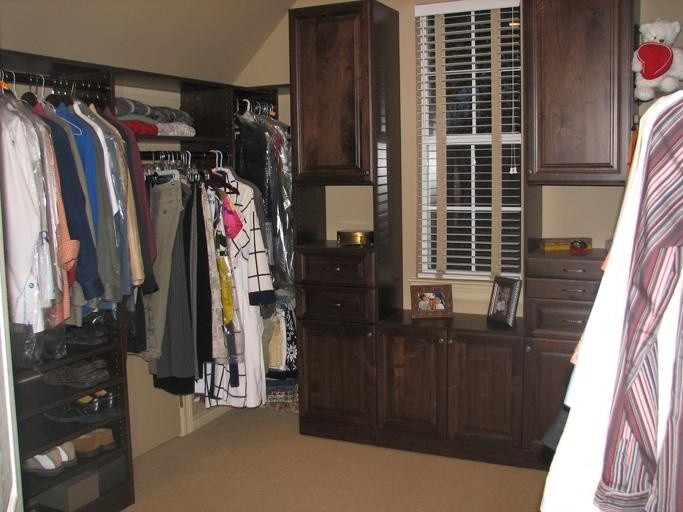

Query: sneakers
<box><xmin>22</xmin><ymin>429</ymin><xmax>116</xmax><ymax>477</ymax></box>
<box><xmin>42</xmin><ymin>358</ymin><xmax>109</xmax><ymax>389</ymax></box>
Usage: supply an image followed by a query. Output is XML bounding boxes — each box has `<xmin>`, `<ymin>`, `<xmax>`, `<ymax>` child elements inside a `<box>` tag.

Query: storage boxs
<box><xmin>37</xmin><ymin>473</ymin><xmax>99</xmax><ymax>512</ymax></box>
<box><xmin>87</xmin><ymin>454</ymin><xmax>130</xmax><ymax>495</ymax></box>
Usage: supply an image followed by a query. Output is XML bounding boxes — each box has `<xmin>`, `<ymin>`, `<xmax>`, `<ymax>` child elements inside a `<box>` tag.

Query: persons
<box><xmin>495</xmin><ymin>291</ymin><xmax>506</xmax><ymax>315</ymax></box>
<box><xmin>418</xmin><ymin>292</ymin><xmax>444</xmax><ymax>312</ymax></box>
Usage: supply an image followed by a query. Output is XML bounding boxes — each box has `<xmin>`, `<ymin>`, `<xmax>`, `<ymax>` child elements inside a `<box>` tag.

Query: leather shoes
<box><xmin>43</xmin><ymin>388</ymin><xmax>121</xmax><ymax>424</ymax></box>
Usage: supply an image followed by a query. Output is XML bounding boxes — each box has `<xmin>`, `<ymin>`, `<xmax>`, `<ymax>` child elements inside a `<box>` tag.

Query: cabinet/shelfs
<box><xmin>525</xmin><ymin>249</ymin><xmax>604</xmax><ymax>340</ymax></box>
<box><xmin>288</xmin><ymin>1</ymin><xmax>403</xmax><ymax>325</ymax></box>
<box><xmin>378</xmin><ymin>325</ymin><xmax>523</xmax><ymax>466</ymax></box>
<box><xmin>522</xmin><ymin>1</ymin><xmax>639</xmax><ymax>186</ymax></box>
<box><xmin>523</xmin><ymin>337</ymin><xmax>576</xmax><ymax>471</ymax></box>
<box><xmin>297</xmin><ymin>321</ymin><xmax>379</xmax><ymax>441</ymax></box>
<box><xmin>0</xmin><ymin>49</ymin><xmax>290</xmax><ymax>512</ymax></box>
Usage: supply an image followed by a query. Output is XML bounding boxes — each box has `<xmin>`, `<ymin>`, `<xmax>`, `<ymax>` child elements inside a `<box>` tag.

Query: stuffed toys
<box><xmin>630</xmin><ymin>18</ymin><xmax>682</xmax><ymax>103</ymax></box>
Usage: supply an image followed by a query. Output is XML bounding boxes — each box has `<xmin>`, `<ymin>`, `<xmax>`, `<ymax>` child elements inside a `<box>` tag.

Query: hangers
<box><xmin>143</xmin><ymin>150</ymin><xmax>263</xmax><ymax>195</ymax></box>
<box><xmin>232</xmin><ymin>98</ymin><xmax>290</xmax><ymax>144</ymax></box>
<box><xmin>0</xmin><ymin>69</ymin><xmax>136</xmax><ymax>140</ymax></box>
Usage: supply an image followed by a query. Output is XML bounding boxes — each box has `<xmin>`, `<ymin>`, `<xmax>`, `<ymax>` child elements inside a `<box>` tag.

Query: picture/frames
<box><xmin>410</xmin><ymin>284</ymin><xmax>453</xmax><ymax>318</ymax></box>
<box><xmin>487</xmin><ymin>276</ymin><xmax>522</xmax><ymax>328</ymax></box>
